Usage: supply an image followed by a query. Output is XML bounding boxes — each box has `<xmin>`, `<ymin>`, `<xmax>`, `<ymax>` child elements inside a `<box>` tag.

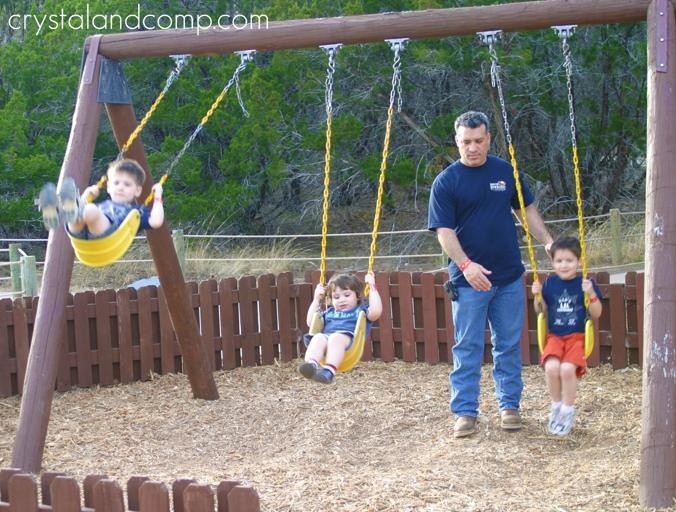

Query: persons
<box><xmin>531</xmin><ymin>235</ymin><xmax>603</xmax><ymax>438</ymax></box>
<box><xmin>428</xmin><ymin>110</ymin><xmax>554</xmax><ymax>439</ymax></box>
<box><xmin>38</xmin><ymin>158</ymin><xmax>165</xmax><ymax>240</ymax></box>
<box><xmin>299</xmin><ymin>271</ymin><xmax>384</xmax><ymax>383</ymax></box>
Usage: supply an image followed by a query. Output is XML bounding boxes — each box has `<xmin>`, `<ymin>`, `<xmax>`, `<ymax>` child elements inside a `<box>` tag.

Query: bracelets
<box><xmin>589</xmin><ymin>295</ymin><xmax>599</xmax><ymax>304</ymax></box>
<box><xmin>370</xmin><ymin>284</ymin><xmax>376</xmax><ymax>289</ymax></box>
<box><xmin>459</xmin><ymin>258</ymin><xmax>471</xmax><ymax>272</ymax></box>
<box><xmin>153</xmin><ymin>198</ymin><xmax>163</xmax><ymax>205</ymax></box>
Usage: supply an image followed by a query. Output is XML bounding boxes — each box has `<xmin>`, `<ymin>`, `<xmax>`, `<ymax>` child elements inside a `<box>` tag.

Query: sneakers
<box><xmin>314</xmin><ymin>366</ymin><xmax>335</xmax><ymax>383</ymax></box>
<box><xmin>555</xmin><ymin>407</ymin><xmax>575</xmax><ymax>434</ymax></box>
<box><xmin>39</xmin><ymin>182</ymin><xmax>60</xmax><ymax>232</ymax></box>
<box><xmin>548</xmin><ymin>402</ymin><xmax>562</xmax><ymax>434</ymax></box>
<box><xmin>59</xmin><ymin>177</ymin><xmax>81</xmax><ymax>225</ymax></box>
<box><xmin>299</xmin><ymin>359</ymin><xmax>319</xmax><ymax>379</ymax></box>
<box><xmin>455</xmin><ymin>415</ymin><xmax>476</xmax><ymax>437</ymax></box>
<box><xmin>501</xmin><ymin>410</ymin><xmax>521</xmax><ymax>430</ymax></box>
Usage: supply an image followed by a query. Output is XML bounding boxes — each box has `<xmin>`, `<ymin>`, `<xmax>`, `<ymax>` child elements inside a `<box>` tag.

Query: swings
<box><xmin>478</xmin><ymin>24</ymin><xmax>595</xmax><ymax>361</ymax></box>
<box><xmin>306</xmin><ymin>38</ymin><xmax>406</xmax><ymax>375</ymax></box>
<box><xmin>62</xmin><ymin>47</ymin><xmax>255</xmax><ymax>266</ymax></box>
